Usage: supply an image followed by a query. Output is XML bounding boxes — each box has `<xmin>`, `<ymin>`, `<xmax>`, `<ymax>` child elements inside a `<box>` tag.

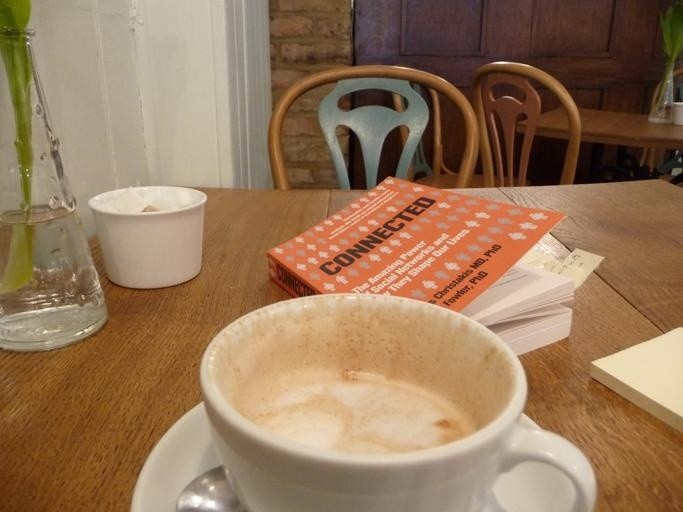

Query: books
<box><xmin>588</xmin><ymin>326</ymin><xmax>682</xmax><ymax>430</ymax></box>
<box><xmin>267</xmin><ymin>175</ymin><xmax>575</xmax><ymax>363</ymax></box>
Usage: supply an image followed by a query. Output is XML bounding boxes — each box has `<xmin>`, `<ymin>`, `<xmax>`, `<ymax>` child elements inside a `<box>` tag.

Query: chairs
<box><xmin>265</xmin><ymin>56</ymin><xmax>578</xmax><ymax>190</ymax></box>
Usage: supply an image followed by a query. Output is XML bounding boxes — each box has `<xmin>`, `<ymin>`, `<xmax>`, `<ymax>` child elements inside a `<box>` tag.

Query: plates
<box><xmin>128</xmin><ymin>388</ymin><xmax>595</xmax><ymax>512</ymax></box>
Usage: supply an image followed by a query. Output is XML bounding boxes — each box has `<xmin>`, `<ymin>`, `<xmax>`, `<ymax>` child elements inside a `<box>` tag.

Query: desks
<box><xmin>512</xmin><ymin>106</ymin><xmax>683</xmax><ymax>182</ymax></box>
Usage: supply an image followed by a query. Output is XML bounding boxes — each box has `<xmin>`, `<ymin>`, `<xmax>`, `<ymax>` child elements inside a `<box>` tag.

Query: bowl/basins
<box><xmin>88</xmin><ymin>183</ymin><xmax>208</xmax><ymax>295</ymax></box>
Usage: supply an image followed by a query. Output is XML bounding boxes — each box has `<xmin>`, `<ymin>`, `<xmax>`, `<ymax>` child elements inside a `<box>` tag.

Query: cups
<box><xmin>199</xmin><ymin>290</ymin><xmax>599</xmax><ymax>512</ymax></box>
<box><xmin>672</xmin><ymin>100</ymin><xmax>682</xmax><ymax>127</ymax></box>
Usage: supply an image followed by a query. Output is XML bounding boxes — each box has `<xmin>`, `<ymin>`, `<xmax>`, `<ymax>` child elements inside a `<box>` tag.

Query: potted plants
<box><xmin>0</xmin><ymin>0</ymin><xmax>112</xmax><ymax>354</ymax></box>
<box><xmin>644</xmin><ymin>0</ymin><xmax>683</xmax><ymax>123</ymax></box>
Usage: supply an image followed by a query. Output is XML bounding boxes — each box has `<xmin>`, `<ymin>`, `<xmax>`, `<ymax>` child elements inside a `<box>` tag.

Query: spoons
<box><xmin>174</xmin><ymin>463</ymin><xmax>241</xmax><ymax>512</ymax></box>
